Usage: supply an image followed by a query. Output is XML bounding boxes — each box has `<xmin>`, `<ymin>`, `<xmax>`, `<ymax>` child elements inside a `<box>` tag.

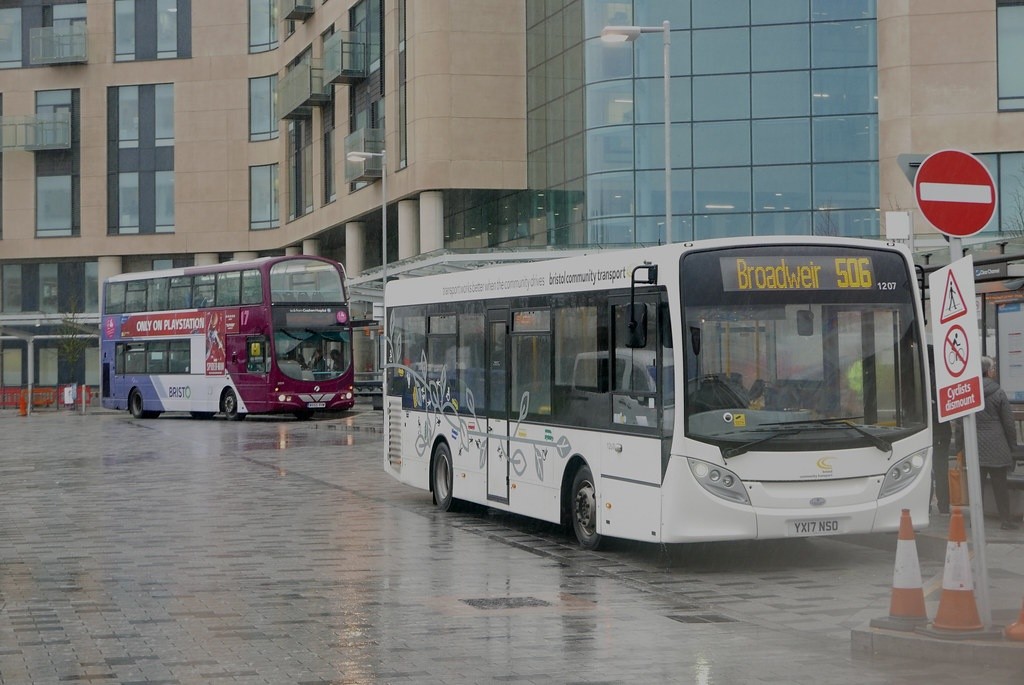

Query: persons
<box><xmin>928</xmin><ymin>344</ymin><xmax>951</xmax><ymax>515</ymax></box>
<box><xmin>955</xmin><ymin>357</ymin><xmax>1020</xmax><ymax>531</ymax></box>
<box><xmin>307</xmin><ymin>348</ymin><xmax>326</xmax><ymax>380</ymax></box>
<box><xmin>330</xmin><ymin>349</ymin><xmax>344</xmax><ymax>377</ymax></box>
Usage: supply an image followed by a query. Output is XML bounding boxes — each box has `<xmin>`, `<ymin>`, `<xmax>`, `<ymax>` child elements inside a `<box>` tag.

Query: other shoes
<box><xmin>1001</xmin><ymin>521</ymin><xmax>1019</xmax><ymax>530</ymax></box>
<box><xmin>929</xmin><ymin>505</ymin><xmax>932</xmax><ymax>516</ymax></box>
<box><xmin>940</xmin><ymin>505</ymin><xmax>950</xmax><ymax>516</ymax></box>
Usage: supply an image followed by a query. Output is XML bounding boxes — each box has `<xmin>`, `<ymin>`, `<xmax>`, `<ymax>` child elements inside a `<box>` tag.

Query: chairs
<box><xmin>271</xmin><ymin>291</ymin><xmax>281</xmax><ymax>303</ymax></box>
<box><xmin>299</xmin><ymin>291</ymin><xmax>311</xmax><ymax>302</ymax></box>
<box><xmin>283</xmin><ymin>290</ymin><xmax>296</xmax><ymax>303</ymax></box>
<box><xmin>312</xmin><ymin>291</ymin><xmax>326</xmax><ymax>303</ymax></box>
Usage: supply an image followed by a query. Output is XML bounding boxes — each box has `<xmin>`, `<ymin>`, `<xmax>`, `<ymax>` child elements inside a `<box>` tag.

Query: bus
<box><xmin>382</xmin><ymin>234</ymin><xmax>935</xmax><ymax>551</ymax></box>
<box><xmin>97</xmin><ymin>255</ymin><xmax>355</xmax><ymax>421</ymax></box>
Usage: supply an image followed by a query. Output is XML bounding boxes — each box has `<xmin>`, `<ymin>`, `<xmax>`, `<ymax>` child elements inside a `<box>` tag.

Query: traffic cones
<box><xmin>912</xmin><ymin>508</ymin><xmax>1001</xmax><ymax>642</ymax></box>
<box><xmin>869</xmin><ymin>509</ymin><xmax>941</xmax><ymax>636</ymax></box>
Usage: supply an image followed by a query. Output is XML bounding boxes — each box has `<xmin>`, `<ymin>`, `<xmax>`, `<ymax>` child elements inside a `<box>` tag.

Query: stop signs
<box><xmin>913</xmin><ymin>150</ymin><xmax>1006</xmax><ymax>252</ymax></box>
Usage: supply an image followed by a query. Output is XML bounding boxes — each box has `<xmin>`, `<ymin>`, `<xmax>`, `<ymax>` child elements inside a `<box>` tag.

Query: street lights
<box><xmin>598</xmin><ymin>19</ymin><xmax>673</xmax><ymax>245</ymax></box>
